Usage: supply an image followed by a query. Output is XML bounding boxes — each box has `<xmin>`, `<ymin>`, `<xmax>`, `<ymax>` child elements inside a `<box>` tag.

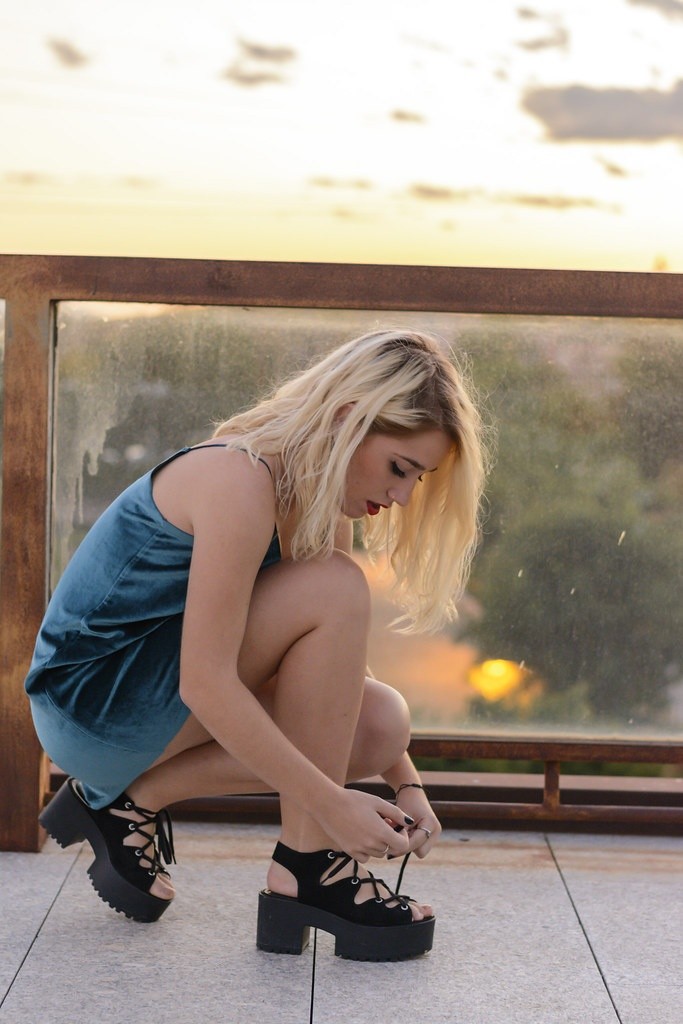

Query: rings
<box><xmin>379</xmin><ymin>844</ymin><xmax>390</xmax><ymax>854</ymax></box>
<box><xmin>416</xmin><ymin>826</ymin><xmax>431</xmax><ymax>839</ymax></box>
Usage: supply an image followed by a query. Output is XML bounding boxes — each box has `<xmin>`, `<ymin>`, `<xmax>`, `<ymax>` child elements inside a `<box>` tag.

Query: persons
<box><xmin>22</xmin><ymin>324</ymin><xmax>492</xmax><ymax>962</ymax></box>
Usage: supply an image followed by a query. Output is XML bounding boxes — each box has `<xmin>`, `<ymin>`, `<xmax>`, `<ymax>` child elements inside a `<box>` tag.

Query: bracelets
<box><xmin>393</xmin><ymin>783</ymin><xmax>431</xmax><ymax>804</ymax></box>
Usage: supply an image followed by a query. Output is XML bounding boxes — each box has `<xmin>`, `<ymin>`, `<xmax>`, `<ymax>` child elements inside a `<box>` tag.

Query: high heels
<box><xmin>39</xmin><ymin>774</ymin><xmax>173</xmax><ymax>925</ymax></box>
<box><xmin>254</xmin><ymin>842</ymin><xmax>437</xmax><ymax>962</ymax></box>
